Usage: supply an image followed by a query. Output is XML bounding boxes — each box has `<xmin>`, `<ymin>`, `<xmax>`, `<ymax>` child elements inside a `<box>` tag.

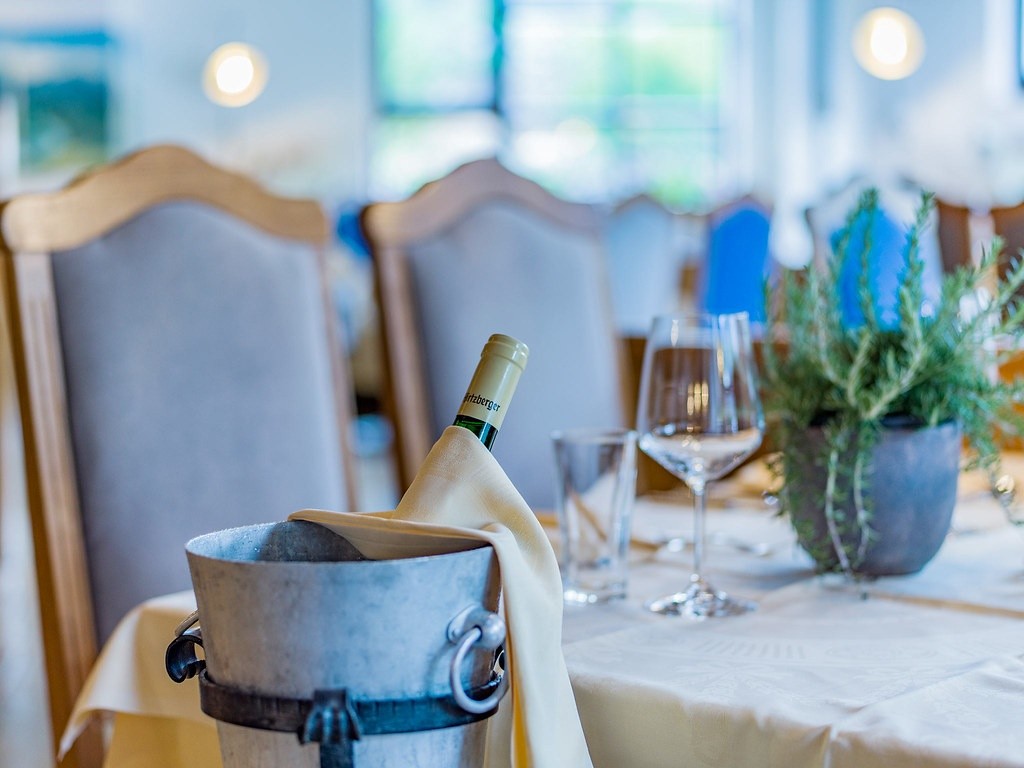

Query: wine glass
<box><xmin>636</xmin><ymin>310</ymin><xmax>766</xmax><ymax>619</ymax></box>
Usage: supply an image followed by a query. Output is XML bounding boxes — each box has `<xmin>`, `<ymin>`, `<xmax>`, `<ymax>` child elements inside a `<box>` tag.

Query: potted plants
<box><xmin>744</xmin><ymin>182</ymin><xmax>1024</xmax><ymax>585</ymax></box>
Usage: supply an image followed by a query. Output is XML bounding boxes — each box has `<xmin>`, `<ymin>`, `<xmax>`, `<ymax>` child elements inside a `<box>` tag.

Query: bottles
<box><xmin>452</xmin><ymin>334</ymin><xmax>529</xmax><ymax>453</ymax></box>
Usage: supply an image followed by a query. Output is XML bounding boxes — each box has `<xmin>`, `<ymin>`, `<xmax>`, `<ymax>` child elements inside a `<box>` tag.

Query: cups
<box><xmin>553</xmin><ymin>429</ymin><xmax>637</xmax><ymax>607</ymax></box>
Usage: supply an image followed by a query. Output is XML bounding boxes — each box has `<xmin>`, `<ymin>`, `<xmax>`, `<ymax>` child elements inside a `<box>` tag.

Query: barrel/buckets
<box><xmin>164</xmin><ymin>521</ymin><xmax>509</xmax><ymax>768</ymax></box>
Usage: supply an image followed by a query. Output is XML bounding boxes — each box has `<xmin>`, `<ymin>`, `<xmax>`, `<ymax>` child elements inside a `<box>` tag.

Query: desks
<box><xmin>60</xmin><ymin>459</ymin><xmax>1024</xmax><ymax>768</ymax></box>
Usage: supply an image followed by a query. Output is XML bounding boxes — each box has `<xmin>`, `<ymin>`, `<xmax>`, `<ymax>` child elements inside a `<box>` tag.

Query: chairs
<box><xmin>0</xmin><ymin>143</ymin><xmax>360</xmax><ymax>768</ymax></box>
<box><xmin>363</xmin><ymin>156</ymin><xmax>634</xmax><ymax>513</ymax></box>
